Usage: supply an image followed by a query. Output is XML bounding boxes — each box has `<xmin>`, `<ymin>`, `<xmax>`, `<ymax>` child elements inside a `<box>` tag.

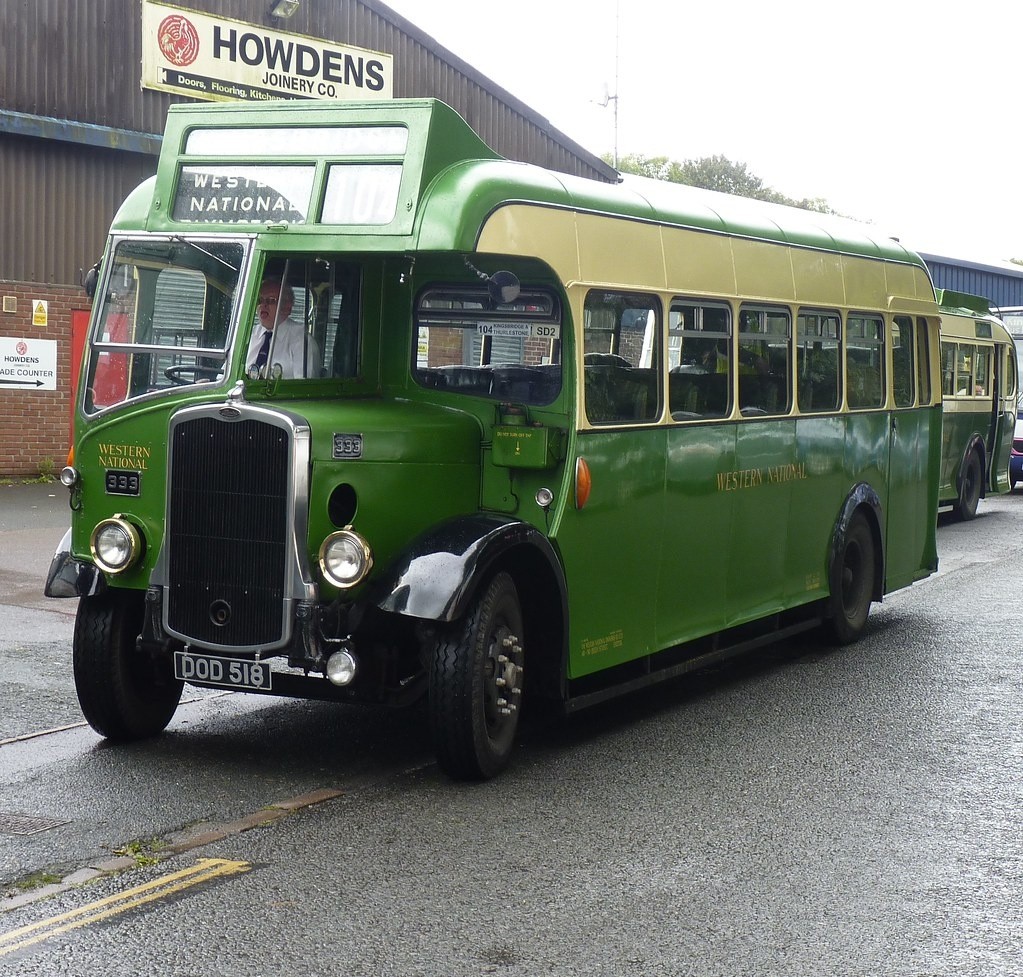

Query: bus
<box><xmin>769</xmin><ymin>286</ymin><xmax>1021</xmax><ymax>523</ymax></box>
<box><xmin>41</xmin><ymin>95</ymin><xmax>946</xmax><ymax>785</ymax></box>
<box><xmin>962</xmin><ymin>306</ymin><xmax>1023</xmax><ymax>490</ymax></box>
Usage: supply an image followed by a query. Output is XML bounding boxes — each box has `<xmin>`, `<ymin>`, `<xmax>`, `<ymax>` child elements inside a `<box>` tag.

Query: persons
<box><xmin>195</xmin><ymin>279</ymin><xmax>321</xmax><ymax>383</ymax></box>
<box><xmin>704</xmin><ymin>312</ymin><xmax>769</xmax><ymax>375</ymax></box>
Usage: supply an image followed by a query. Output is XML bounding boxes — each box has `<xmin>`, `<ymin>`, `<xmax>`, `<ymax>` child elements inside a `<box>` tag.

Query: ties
<box><xmin>256</xmin><ymin>331</ymin><xmax>272</xmax><ymax>368</ymax></box>
